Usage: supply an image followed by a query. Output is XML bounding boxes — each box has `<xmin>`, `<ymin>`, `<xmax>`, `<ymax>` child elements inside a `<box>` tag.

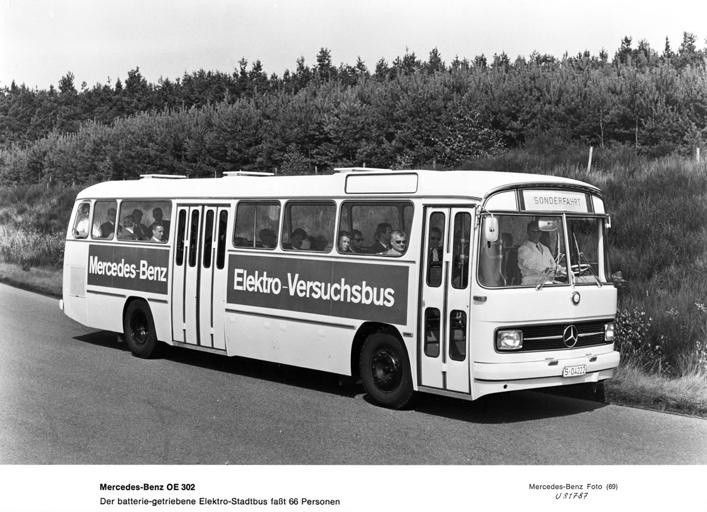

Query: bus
<box><xmin>58</xmin><ymin>165</ymin><xmax>620</xmax><ymax>410</ymax></box>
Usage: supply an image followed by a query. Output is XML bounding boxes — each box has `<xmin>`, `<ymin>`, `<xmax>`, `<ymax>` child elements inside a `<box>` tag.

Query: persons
<box><xmin>290</xmin><ymin>223</ymin><xmax>406</xmax><ymax>256</ymax></box>
<box><xmin>205</xmin><ymin>221</ymin><xmax>226</xmax><ymax>269</ymax></box>
<box><xmin>429</xmin><ymin>221</ymin><xmax>557</xmax><ymax>327</ymax></box>
<box><xmin>74</xmin><ymin>203</ymin><xmax>170</xmax><ymax>243</ymax></box>
<box><xmin>260</xmin><ymin>228</ymin><xmax>277</xmax><ymax>248</ymax></box>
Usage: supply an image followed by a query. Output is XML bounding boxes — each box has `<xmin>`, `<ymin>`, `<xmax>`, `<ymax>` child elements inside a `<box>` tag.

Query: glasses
<box><xmin>391</xmin><ymin>240</ymin><xmax>405</xmax><ymax>244</ymax></box>
